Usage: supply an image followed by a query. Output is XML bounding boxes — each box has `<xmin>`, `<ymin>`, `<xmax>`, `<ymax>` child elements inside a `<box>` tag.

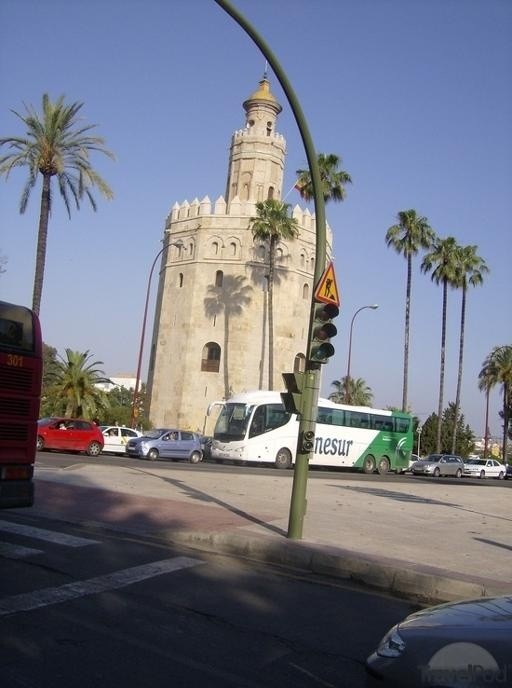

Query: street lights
<box><xmin>343</xmin><ymin>304</ymin><xmax>381</xmax><ymax>408</ymax></box>
<box><xmin>131</xmin><ymin>241</ymin><xmax>187</xmax><ymax>431</ymax></box>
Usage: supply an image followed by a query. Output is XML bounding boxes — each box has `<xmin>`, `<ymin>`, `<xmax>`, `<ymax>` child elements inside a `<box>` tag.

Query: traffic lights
<box><xmin>308</xmin><ymin>301</ymin><xmax>339</xmax><ymax>366</ymax></box>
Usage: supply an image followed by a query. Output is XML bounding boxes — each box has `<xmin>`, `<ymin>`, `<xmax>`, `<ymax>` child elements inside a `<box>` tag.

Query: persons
<box><xmin>109</xmin><ymin>429</ymin><xmax>117</xmax><ymax>436</ymax></box>
<box><xmin>169</xmin><ymin>433</ymin><xmax>176</xmax><ymax>441</ymax></box>
<box><xmin>58</xmin><ymin>420</ymin><xmax>68</xmax><ymax>431</ymax></box>
<box><xmin>92</xmin><ymin>418</ymin><xmax>101</xmax><ymax>426</ymax></box>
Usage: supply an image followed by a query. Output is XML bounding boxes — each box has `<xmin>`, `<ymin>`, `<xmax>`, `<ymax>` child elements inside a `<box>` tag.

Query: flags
<box><xmin>295</xmin><ymin>175</ymin><xmax>314</xmax><ymax>201</ymax></box>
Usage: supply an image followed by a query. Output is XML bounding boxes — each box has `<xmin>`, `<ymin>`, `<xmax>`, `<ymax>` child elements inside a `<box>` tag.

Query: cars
<box><xmin>402</xmin><ymin>453</ymin><xmax>511</xmax><ymax>481</ymax></box>
<box><xmin>37</xmin><ymin>418</ymin><xmax>216</xmax><ymax>465</ymax></box>
<box><xmin>362</xmin><ymin>594</ymin><xmax>511</xmax><ymax>687</ymax></box>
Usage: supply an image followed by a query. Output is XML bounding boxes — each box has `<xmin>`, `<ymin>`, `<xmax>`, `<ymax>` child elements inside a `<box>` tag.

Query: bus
<box><xmin>206</xmin><ymin>391</ymin><xmax>417</xmax><ymax>476</ymax></box>
<box><xmin>0</xmin><ymin>301</ymin><xmax>48</xmax><ymax>512</ymax></box>
